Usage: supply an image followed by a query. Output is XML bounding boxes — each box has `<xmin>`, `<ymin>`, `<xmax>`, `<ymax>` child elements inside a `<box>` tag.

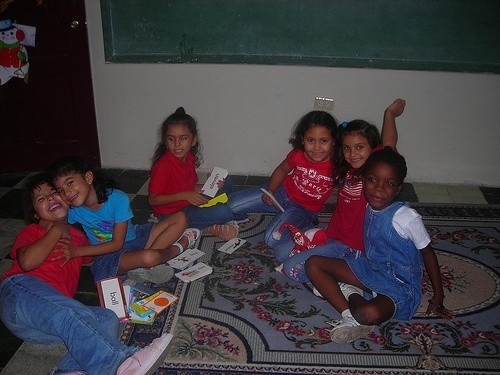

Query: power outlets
<box><xmin>314</xmin><ymin>96</ymin><xmax>335</xmax><ymax>112</ymax></box>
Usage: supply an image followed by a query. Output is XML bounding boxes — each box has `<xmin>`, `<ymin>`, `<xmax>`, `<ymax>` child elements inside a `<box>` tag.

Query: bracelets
<box><xmin>428</xmin><ymin>300</ymin><xmax>444</xmax><ymax>308</ymax></box>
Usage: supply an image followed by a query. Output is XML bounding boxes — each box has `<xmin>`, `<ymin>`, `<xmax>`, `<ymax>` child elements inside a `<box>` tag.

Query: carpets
<box><xmin>113</xmin><ymin>204</ymin><xmax>500</xmax><ymax>375</ymax></box>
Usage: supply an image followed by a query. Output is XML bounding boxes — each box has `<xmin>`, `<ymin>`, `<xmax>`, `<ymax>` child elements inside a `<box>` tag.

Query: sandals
<box><xmin>127</xmin><ymin>264</ymin><xmax>174</xmax><ymax>284</ymax></box>
<box><xmin>173</xmin><ymin>228</ymin><xmax>200</xmax><ymax>253</ymax></box>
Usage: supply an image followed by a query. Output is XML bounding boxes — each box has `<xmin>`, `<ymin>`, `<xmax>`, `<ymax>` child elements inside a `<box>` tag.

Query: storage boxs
<box><xmin>96</xmin><ymin>276</ymin><xmax>163</xmax><ymax>325</ymax></box>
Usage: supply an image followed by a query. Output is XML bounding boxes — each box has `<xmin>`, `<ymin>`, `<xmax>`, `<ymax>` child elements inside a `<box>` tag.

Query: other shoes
<box><xmin>208</xmin><ymin>223</ymin><xmax>239</xmax><ymax>240</ymax></box>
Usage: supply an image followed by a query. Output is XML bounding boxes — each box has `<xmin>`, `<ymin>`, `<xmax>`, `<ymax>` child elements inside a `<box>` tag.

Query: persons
<box><xmin>273</xmin><ymin>98</ymin><xmax>407</xmax><ymax>281</ymax></box>
<box><xmin>0</xmin><ymin>172</ymin><xmax>174</xmax><ymax>375</ymax></box>
<box><xmin>226</xmin><ymin>111</ymin><xmax>337</xmax><ymax>249</ymax></box>
<box><xmin>48</xmin><ymin>155</ymin><xmax>200</xmax><ymax>284</ymax></box>
<box><xmin>147</xmin><ymin>107</ymin><xmax>238</xmax><ymax>241</ymax></box>
<box><xmin>304</xmin><ymin>145</ymin><xmax>456</xmax><ymax>343</ymax></box>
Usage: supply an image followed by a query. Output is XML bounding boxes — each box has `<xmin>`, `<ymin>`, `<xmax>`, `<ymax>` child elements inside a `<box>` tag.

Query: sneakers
<box><xmin>116</xmin><ymin>333</ymin><xmax>174</xmax><ymax>375</ymax></box>
<box><xmin>313</xmin><ymin>281</ymin><xmax>363</xmax><ymax>302</ymax></box>
<box><xmin>330</xmin><ymin>316</ymin><xmax>375</xmax><ymax>344</ymax></box>
